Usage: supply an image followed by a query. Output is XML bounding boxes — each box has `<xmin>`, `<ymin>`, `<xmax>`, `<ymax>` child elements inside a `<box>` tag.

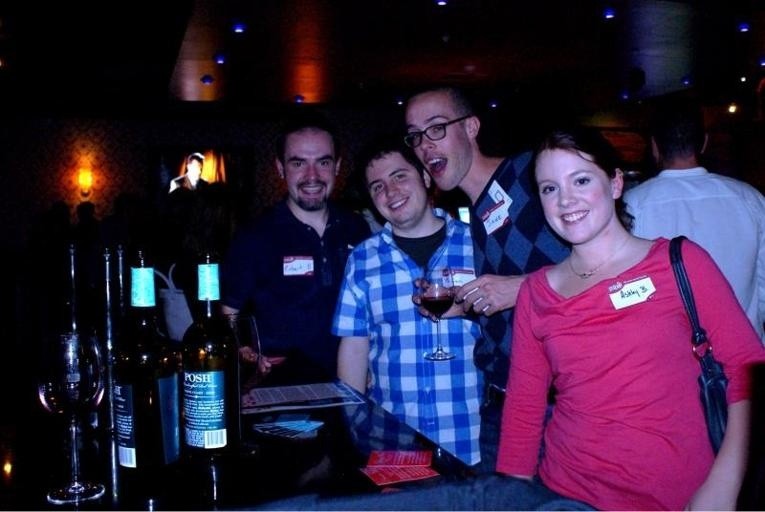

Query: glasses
<box><xmin>402</xmin><ymin>114</ymin><xmax>469</xmax><ymax>148</ymax></box>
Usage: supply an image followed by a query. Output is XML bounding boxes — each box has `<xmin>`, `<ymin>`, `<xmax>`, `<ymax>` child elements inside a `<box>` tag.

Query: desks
<box><xmin>239</xmin><ymin>373</ymin><xmax>483</xmax><ymax>512</ymax></box>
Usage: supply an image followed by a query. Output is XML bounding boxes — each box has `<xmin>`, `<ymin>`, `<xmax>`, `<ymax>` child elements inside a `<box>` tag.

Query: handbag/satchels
<box><xmin>697</xmin><ymin>355</ymin><xmax>727</xmax><ymax>452</ymax></box>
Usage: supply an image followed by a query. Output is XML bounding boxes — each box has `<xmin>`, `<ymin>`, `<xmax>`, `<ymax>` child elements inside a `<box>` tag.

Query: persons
<box><xmin>217</xmin><ymin>117</ymin><xmax>374</xmax><ymax>372</ymax></box>
<box><xmin>622</xmin><ymin>102</ymin><xmax>765</xmax><ymax>344</ymax></box>
<box><xmin>2</xmin><ymin>191</ymin><xmax>158</xmax><ymax>266</ymax></box>
<box><xmin>330</xmin><ymin>142</ymin><xmax>483</xmax><ymax>469</ymax></box>
<box><xmin>169</xmin><ymin>153</ymin><xmax>209</xmax><ymax>189</ymax></box>
<box><xmin>405</xmin><ymin>82</ymin><xmax>575</xmax><ymax>476</ymax></box>
<box><xmin>494</xmin><ymin>125</ymin><xmax>765</xmax><ymax>510</ymax></box>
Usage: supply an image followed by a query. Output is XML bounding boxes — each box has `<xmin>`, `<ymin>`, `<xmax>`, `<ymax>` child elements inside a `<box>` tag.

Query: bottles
<box><xmin>61</xmin><ymin>237</ymin><xmax>248</xmax><ymax>510</ymax></box>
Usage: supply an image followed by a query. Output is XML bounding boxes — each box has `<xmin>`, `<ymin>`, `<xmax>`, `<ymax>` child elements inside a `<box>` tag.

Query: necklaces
<box><xmin>569</xmin><ymin>236</ymin><xmax>635</xmax><ymax>278</ymax></box>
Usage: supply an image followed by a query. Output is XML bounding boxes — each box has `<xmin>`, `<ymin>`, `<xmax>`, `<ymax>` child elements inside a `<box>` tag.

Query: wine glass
<box><xmin>35</xmin><ymin>329</ymin><xmax>110</xmax><ymax>500</ymax></box>
<box><xmin>420</xmin><ymin>268</ymin><xmax>461</xmax><ymax>364</ymax></box>
